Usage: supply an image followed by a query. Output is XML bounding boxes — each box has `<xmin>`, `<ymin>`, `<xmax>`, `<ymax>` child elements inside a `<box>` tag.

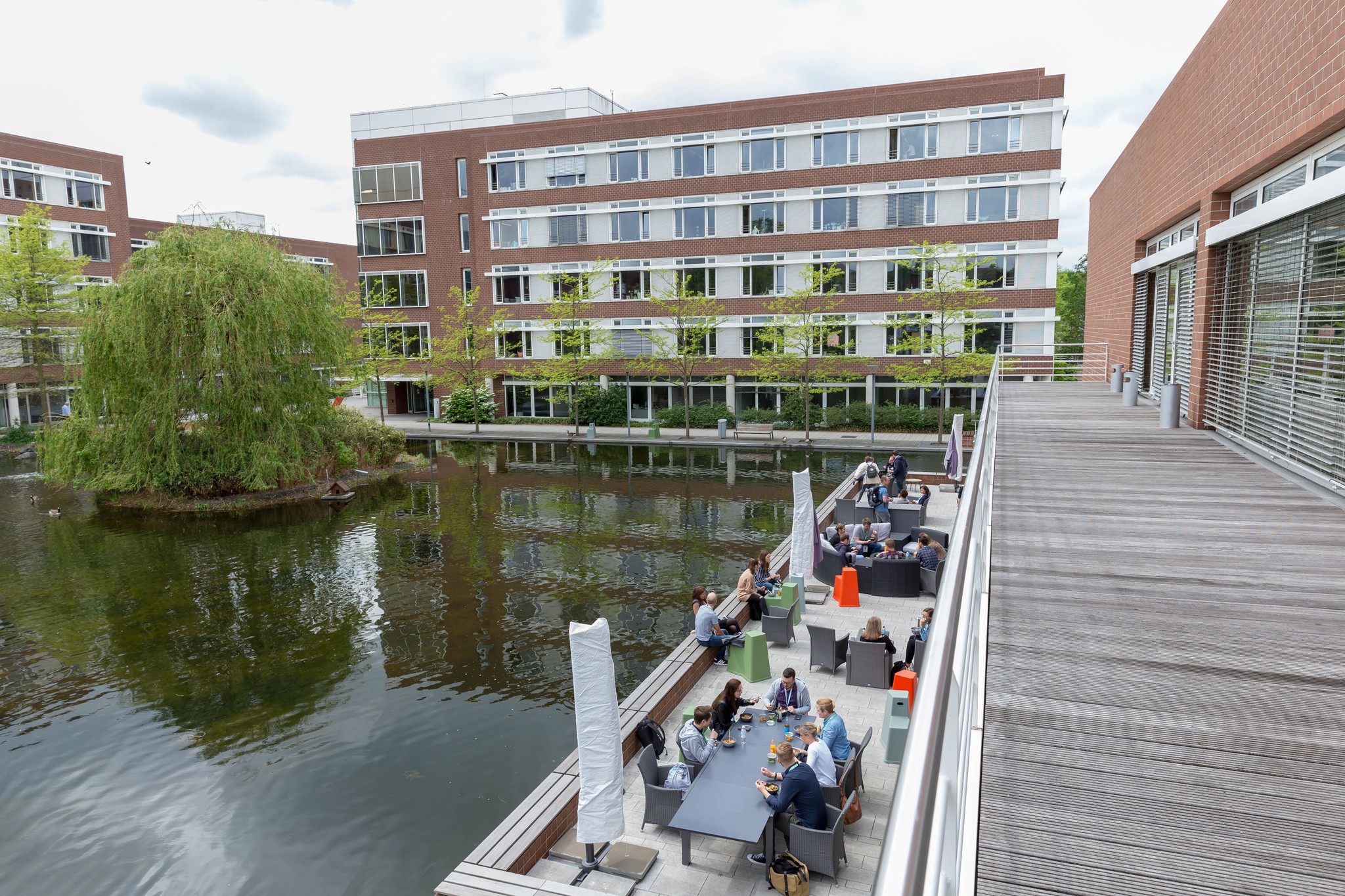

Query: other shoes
<box><xmin>919</xmin><ymin>589</ymin><xmax>924</xmax><ymax>592</ymax></box>
<box><xmin>861</xmin><ymin>489</ymin><xmax>868</xmax><ymax>492</ymax></box>
<box><xmin>910</xmin><ymin>663</ymin><xmax>913</xmax><ymax>668</ymax></box>
<box><xmin>856</xmin><ymin>501</ymin><xmax>859</xmax><ymax>503</ymax></box>
<box><xmin>908</xmin><ymin>667</ymin><xmax>912</xmax><ymax>672</ymax></box>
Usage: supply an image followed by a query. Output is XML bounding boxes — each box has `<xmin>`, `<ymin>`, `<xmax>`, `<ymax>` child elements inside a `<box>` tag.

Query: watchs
<box><xmin>774</xmin><ymin>772</ymin><xmax>777</xmax><ymax>779</ymax></box>
<box><xmin>890</xmin><ymin>499</ymin><xmax>891</xmax><ymax>501</ymax></box>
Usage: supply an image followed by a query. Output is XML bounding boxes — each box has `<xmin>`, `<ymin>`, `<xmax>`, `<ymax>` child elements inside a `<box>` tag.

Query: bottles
<box><xmin>784</xmin><ymin>717</ymin><xmax>790</xmax><ymax>733</ymax></box>
<box><xmin>778</xmin><ymin>584</ymin><xmax>782</xmax><ymax>596</ymax></box>
<box><xmin>884</xmin><ymin>546</ymin><xmax>887</xmax><ymax>552</ymax></box>
<box><xmin>887</xmin><ymin>466</ymin><xmax>890</xmax><ymax>470</ymax></box>
<box><xmin>740</xmin><ymin>726</ymin><xmax>747</xmax><ymax>744</ymax></box>
<box><xmin>863</xmin><ymin>628</ymin><xmax>865</xmax><ymax>632</ymax></box>
<box><xmin>776</xmin><ymin>703</ymin><xmax>782</xmax><ymax>722</ymax></box>
<box><xmin>906</xmin><ymin>550</ymin><xmax>910</xmax><ymax>559</ymax></box>
<box><xmin>882</xmin><ymin>626</ymin><xmax>886</xmax><ymax>634</ymax></box>
<box><xmin>770</xmin><ymin>739</ymin><xmax>776</xmax><ymax>754</ymax></box>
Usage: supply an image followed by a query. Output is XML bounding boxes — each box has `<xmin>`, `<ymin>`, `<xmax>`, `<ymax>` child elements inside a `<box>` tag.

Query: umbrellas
<box><xmin>793</xmin><ymin>467</ymin><xmax>824</xmax><ymax>569</ymax></box>
<box><xmin>946</xmin><ymin>414</ymin><xmax>964</xmax><ymax>484</ymax></box>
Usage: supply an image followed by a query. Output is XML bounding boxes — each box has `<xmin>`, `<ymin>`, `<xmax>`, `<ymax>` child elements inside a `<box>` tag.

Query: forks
<box><xmin>747</xmin><ymin>698</ymin><xmax>758</xmax><ymax>703</ymax></box>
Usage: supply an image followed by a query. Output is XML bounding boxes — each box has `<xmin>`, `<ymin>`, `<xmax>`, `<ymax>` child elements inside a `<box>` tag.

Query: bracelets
<box><xmin>767</xmin><ymin>577</ymin><xmax>769</xmax><ymax>581</ymax></box>
<box><xmin>770</xmin><ymin>576</ymin><xmax>772</xmax><ymax>579</ymax></box>
<box><xmin>713</xmin><ymin>738</ymin><xmax>716</xmax><ymax>740</ymax></box>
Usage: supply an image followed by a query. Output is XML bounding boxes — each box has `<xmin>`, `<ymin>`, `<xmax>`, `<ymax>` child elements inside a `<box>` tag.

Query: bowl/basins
<box><xmin>764</xmin><ymin>783</ymin><xmax>779</xmax><ymax>794</ymax></box>
<box><xmin>767</xmin><ymin>713</ymin><xmax>776</xmax><ymax>721</ymax></box>
<box><xmin>767</xmin><ymin>753</ymin><xmax>777</xmax><ymax>763</ymax></box>
<box><xmin>773</xmin><ymin>707</ymin><xmax>788</xmax><ymax>716</ymax></box>
<box><xmin>768</xmin><ymin>591</ymin><xmax>777</xmax><ymax>596</ymax></box>
<box><xmin>740</xmin><ymin>713</ymin><xmax>753</xmax><ymax>722</ymax></box>
<box><xmin>793</xmin><ymin>725</ymin><xmax>800</xmax><ymax>734</ymax></box>
<box><xmin>784</xmin><ymin>732</ymin><xmax>794</xmax><ymax>741</ymax></box>
<box><xmin>774</xmin><ymin>583</ymin><xmax>783</xmax><ymax>587</ymax></box>
<box><xmin>793</xmin><ymin>748</ymin><xmax>799</xmax><ymax>757</ymax></box>
<box><xmin>721</xmin><ymin>737</ymin><xmax>736</xmax><ymax>747</ymax></box>
<box><xmin>759</xmin><ymin>714</ymin><xmax>767</xmax><ymax>723</ymax></box>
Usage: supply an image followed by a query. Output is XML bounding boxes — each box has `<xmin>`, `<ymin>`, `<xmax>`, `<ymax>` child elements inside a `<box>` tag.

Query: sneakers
<box><xmin>747</xmin><ymin>852</ymin><xmax>766</xmax><ymax>866</ymax></box>
<box><xmin>713</xmin><ymin>657</ymin><xmax>728</xmax><ymax>665</ymax></box>
<box><xmin>775</xmin><ymin>848</ymin><xmax>789</xmax><ymax>859</ymax></box>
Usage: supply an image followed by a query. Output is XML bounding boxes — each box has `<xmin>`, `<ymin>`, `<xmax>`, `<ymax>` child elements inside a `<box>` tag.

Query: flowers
<box><xmin>628</xmin><ymin>290</ymin><xmax>633</xmax><ymax>298</ymax></box>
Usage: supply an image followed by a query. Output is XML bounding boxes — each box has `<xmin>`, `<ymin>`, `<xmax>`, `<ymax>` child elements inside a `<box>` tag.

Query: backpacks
<box><xmin>867</xmin><ymin>484</ymin><xmax>889</xmax><ymax>507</ymax></box>
<box><xmin>664</xmin><ymin>762</ymin><xmax>692</xmax><ymax>792</ymax></box>
<box><xmin>867</xmin><ymin>463</ymin><xmax>878</xmax><ymax>478</ymax></box>
<box><xmin>635</xmin><ymin>717</ymin><xmax>668</xmax><ymax>759</ymax></box>
<box><xmin>891</xmin><ymin>660</ymin><xmax>910</xmax><ymax>688</ymax></box>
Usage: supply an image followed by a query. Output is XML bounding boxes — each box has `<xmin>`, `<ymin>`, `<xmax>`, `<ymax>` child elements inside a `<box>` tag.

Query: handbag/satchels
<box><xmin>910</xmin><ymin>501</ymin><xmax>916</xmax><ymax>504</ymax></box>
<box><xmin>768</xmin><ymin>852</ymin><xmax>810</xmax><ymax>896</ymax></box>
<box><xmin>722</xmin><ymin>632</ymin><xmax>746</xmax><ymax>647</ymax></box>
<box><xmin>838</xmin><ymin>759</ymin><xmax>862</xmax><ymax>825</ymax></box>
<box><xmin>749</xmin><ymin>602</ymin><xmax>762</xmax><ymax>621</ymax></box>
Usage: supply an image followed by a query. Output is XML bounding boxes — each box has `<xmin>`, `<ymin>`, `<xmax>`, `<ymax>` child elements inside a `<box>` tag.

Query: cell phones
<box><xmin>794</xmin><ymin>715</ymin><xmax>802</xmax><ymax>720</ymax></box>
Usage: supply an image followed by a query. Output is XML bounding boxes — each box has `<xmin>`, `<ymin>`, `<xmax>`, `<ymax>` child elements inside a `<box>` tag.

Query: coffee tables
<box><xmin>870</xmin><ymin>551</ymin><xmax>914</xmax><ymax>559</ymax></box>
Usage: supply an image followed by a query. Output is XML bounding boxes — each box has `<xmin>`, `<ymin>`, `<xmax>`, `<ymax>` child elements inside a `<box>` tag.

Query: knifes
<box><xmin>740</xmin><ymin>724</ymin><xmax>758</xmax><ymax>727</ymax></box>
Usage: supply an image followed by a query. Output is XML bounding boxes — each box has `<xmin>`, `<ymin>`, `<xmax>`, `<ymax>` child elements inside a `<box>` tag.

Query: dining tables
<box><xmin>669</xmin><ymin>708</ymin><xmax>816</xmax><ymax>881</ymax></box>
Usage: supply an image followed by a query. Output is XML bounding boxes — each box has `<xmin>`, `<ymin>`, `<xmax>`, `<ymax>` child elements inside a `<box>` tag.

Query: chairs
<box><xmin>637</xmin><ymin>473</ymin><xmax>949</xmax><ymax>889</ymax></box>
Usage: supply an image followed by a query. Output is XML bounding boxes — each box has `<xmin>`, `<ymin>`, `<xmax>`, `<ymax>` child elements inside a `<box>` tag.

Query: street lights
<box><xmin>866</xmin><ymin>365</ymin><xmax>881</xmax><ymax>443</ymax></box>
<box><xmin>621</xmin><ymin>364</ymin><xmax>632</xmax><ymax>437</ymax></box>
<box><xmin>420</xmin><ymin>364</ymin><xmax>432</xmax><ymax>431</ymax></box>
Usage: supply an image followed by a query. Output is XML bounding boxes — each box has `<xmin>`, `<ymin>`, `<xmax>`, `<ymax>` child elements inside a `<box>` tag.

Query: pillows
<box><xmin>504</xmin><ymin>297</ymin><xmax>521</xmax><ymax>303</ymax></box>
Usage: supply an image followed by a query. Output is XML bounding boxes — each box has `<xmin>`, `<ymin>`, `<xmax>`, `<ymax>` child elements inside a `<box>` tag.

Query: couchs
<box><xmin>812</xmin><ymin>522</ymin><xmax>920</xmax><ymax>598</ymax></box>
<box><xmin>903</xmin><ymin>526</ymin><xmax>949</xmax><ymax>554</ymax></box>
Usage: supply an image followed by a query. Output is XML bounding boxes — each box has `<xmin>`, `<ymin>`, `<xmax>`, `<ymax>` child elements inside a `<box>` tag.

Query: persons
<box><xmin>850</xmin><ymin>517</ymin><xmax>881</xmax><ymax>555</ymax></box>
<box><xmin>854</xmin><ymin>450</ymin><xmax>931</xmax><ymax>506</ymax></box>
<box><xmin>679</xmin><ymin>705</ymin><xmax>718</xmax><ymax>764</ymax></box>
<box><xmin>913</xmin><ymin>532</ymin><xmax>947</xmax><ymax>592</ymax></box>
<box><xmin>905</xmin><ymin>607</ymin><xmax>934</xmax><ymax>667</ymax></box>
<box><xmin>763</xmin><ymin>668</ymin><xmax>811</xmax><ymax>715</ymax></box>
<box><xmin>754</xmin><ymin>550</ymin><xmax>781</xmax><ymax>592</ymax></box>
<box><xmin>816</xmin><ymin>698</ymin><xmax>851</xmax><ymax>760</ymax></box>
<box><xmin>876</xmin><ymin>538</ymin><xmax>906</xmax><ymax>559</ymax></box>
<box><xmin>709</xmin><ymin>678</ymin><xmax>761</xmax><ymax>741</ymax></box>
<box><xmin>695</xmin><ymin>593</ymin><xmax>734</xmax><ymax>665</ymax></box>
<box><xmin>792</xmin><ymin>722</ymin><xmax>836</xmax><ymax>786</ymax></box>
<box><xmin>747</xmin><ymin>741</ymin><xmax>828</xmax><ymax>866</ymax></box>
<box><xmin>875</xmin><ymin>475</ymin><xmax>894</xmax><ymax>538</ymax></box>
<box><xmin>860</xmin><ymin>617</ymin><xmax>896</xmax><ymax>654</ymax></box>
<box><xmin>690</xmin><ymin>586</ymin><xmax>742</xmax><ymax>635</ymax></box>
<box><xmin>737</xmin><ymin>558</ymin><xmax>770</xmax><ymax>616</ymax></box>
<box><xmin>829</xmin><ymin>523</ymin><xmax>864</xmax><ymax>567</ymax></box>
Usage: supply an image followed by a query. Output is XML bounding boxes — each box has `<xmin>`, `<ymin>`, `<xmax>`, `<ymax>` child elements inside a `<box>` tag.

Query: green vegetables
<box><xmin>768</xmin><ymin>715</ymin><xmax>775</xmax><ymax>720</ymax></box>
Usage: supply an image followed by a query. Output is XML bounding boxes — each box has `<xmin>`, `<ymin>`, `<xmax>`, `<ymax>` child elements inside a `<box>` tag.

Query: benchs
<box><xmin>734</xmin><ymin>423</ymin><xmax>774</xmax><ymax>441</ymax></box>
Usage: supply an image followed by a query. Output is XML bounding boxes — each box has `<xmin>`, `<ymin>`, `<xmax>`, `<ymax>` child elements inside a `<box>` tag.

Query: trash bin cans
<box><xmin>1110</xmin><ymin>363</ymin><xmax>1125</xmax><ymax>394</ymax></box>
<box><xmin>717</xmin><ymin>418</ymin><xmax>727</xmax><ymax>439</ymax></box>
<box><xmin>1160</xmin><ymin>383</ymin><xmax>1181</xmax><ymax>429</ymax></box>
<box><xmin>1122</xmin><ymin>372</ymin><xmax>1139</xmax><ymax>406</ymax></box>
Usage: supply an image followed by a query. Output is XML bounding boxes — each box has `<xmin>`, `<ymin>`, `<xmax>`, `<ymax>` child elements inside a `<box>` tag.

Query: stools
<box><xmin>906</xmin><ymin>479</ymin><xmax>922</xmax><ymax>493</ymax></box>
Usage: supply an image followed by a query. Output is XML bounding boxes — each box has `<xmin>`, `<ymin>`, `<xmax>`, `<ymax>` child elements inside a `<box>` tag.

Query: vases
<box><xmin>630</xmin><ymin>293</ymin><xmax>633</xmax><ymax>299</ymax></box>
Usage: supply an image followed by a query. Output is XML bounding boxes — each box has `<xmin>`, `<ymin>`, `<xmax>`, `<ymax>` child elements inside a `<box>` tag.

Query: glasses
<box><xmin>736</xmin><ymin>687</ymin><xmax>743</xmax><ymax>693</ymax></box>
<box><xmin>781</xmin><ymin>677</ymin><xmax>793</xmax><ymax>686</ymax></box>
<box><xmin>921</xmin><ymin>612</ymin><xmax>930</xmax><ymax>617</ymax></box>
<box><xmin>889</xmin><ymin>459</ymin><xmax>894</xmax><ymax>461</ymax></box>
